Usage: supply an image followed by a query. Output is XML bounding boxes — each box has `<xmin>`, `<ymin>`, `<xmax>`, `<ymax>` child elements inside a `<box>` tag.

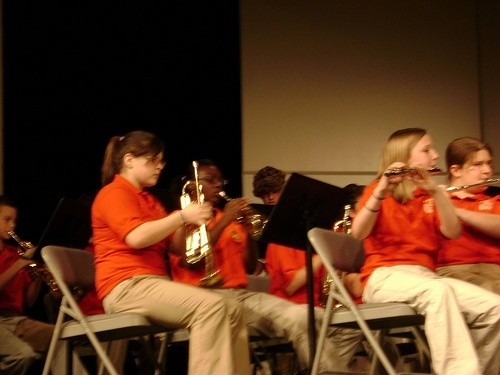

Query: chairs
<box><xmin>308</xmin><ymin>228</ymin><xmax>416</xmax><ymax>375</ymax></box>
<box><xmin>42</xmin><ymin>246</ymin><xmax>293</xmax><ymax>375</ymax></box>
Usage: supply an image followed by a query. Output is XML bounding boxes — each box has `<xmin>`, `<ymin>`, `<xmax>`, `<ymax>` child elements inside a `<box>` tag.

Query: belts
<box><xmin>0</xmin><ymin>310</ymin><xmax>24</xmax><ymax>317</ymax></box>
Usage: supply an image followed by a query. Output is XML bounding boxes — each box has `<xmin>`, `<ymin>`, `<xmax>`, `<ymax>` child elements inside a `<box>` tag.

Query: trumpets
<box><xmin>219</xmin><ymin>193</ymin><xmax>269</xmax><ymax>236</ymax></box>
<box><xmin>319</xmin><ymin>204</ymin><xmax>357</xmax><ymax>307</ymax></box>
<box><xmin>181</xmin><ymin>159</ymin><xmax>225</xmax><ymax>290</ymax></box>
<box><xmin>8</xmin><ymin>230</ymin><xmax>83</xmax><ymax>303</ymax></box>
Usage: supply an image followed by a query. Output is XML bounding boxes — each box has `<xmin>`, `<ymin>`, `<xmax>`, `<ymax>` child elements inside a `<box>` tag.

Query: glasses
<box><xmin>198</xmin><ymin>176</ymin><xmax>229</xmax><ymax>185</ymax></box>
<box><xmin>136</xmin><ymin>156</ymin><xmax>166</xmax><ymax>167</ymax></box>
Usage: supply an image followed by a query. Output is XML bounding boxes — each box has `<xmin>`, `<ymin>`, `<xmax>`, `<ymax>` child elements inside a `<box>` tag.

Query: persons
<box><xmin>434</xmin><ymin>136</ymin><xmax>500</xmax><ymax>296</ymax></box>
<box><xmin>93</xmin><ymin>131</ymin><xmax>250</xmax><ymax>375</ymax></box>
<box><xmin>253</xmin><ymin>166</ymin><xmax>286</xmax><ymax>204</ymax></box>
<box><xmin>343</xmin><ymin>187</ymin><xmax>411</xmax><ymax>375</ymax></box>
<box><xmin>353</xmin><ymin>128</ymin><xmax>500</xmax><ymax>375</ymax></box>
<box><xmin>0</xmin><ymin>195</ymin><xmax>90</xmax><ymax>375</ymax></box>
<box><xmin>74</xmin><ymin>235</ymin><xmax>153</xmax><ymax>375</ymax></box>
<box><xmin>265</xmin><ymin>241</ymin><xmax>402</xmax><ymax>375</ymax></box>
<box><xmin>171</xmin><ymin>157</ymin><xmax>362</xmax><ymax>375</ymax></box>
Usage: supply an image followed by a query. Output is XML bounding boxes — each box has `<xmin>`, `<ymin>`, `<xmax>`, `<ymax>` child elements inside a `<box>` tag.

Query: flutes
<box><xmin>421</xmin><ymin>176</ymin><xmax>499</xmax><ymax>208</ymax></box>
<box><xmin>383</xmin><ymin>168</ymin><xmax>447</xmax><ymax>177</ymax></box>
